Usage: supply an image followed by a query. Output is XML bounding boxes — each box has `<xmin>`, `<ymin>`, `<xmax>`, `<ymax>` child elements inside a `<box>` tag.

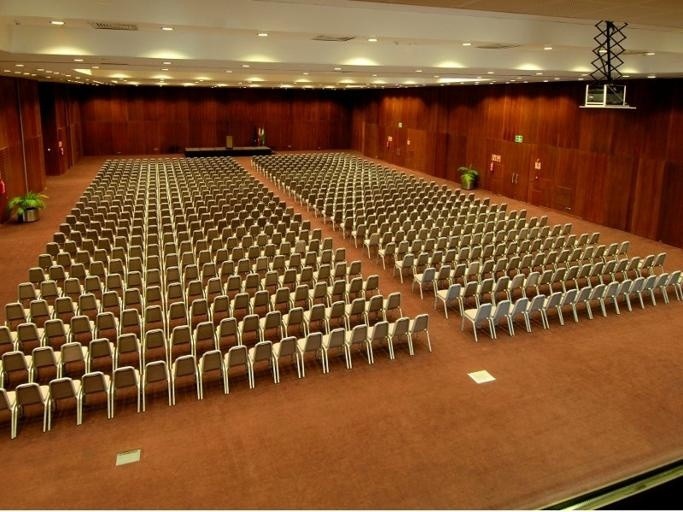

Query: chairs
<box><xmin>0</xmin><ymin>151</ymin><xmax>680</xmax><ymax>438</ymax></box>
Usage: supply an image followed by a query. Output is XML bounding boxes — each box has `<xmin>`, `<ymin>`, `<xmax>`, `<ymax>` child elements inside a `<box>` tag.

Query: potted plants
<box><xmin>7</xmin><ymin>192</ymin><xmax>50</xmax><ymax>223</ymax></box>
<box><xmin>455</xmin><ymin>164</ymin><xmax>478</xmax><ymax>190</ymax></box>
